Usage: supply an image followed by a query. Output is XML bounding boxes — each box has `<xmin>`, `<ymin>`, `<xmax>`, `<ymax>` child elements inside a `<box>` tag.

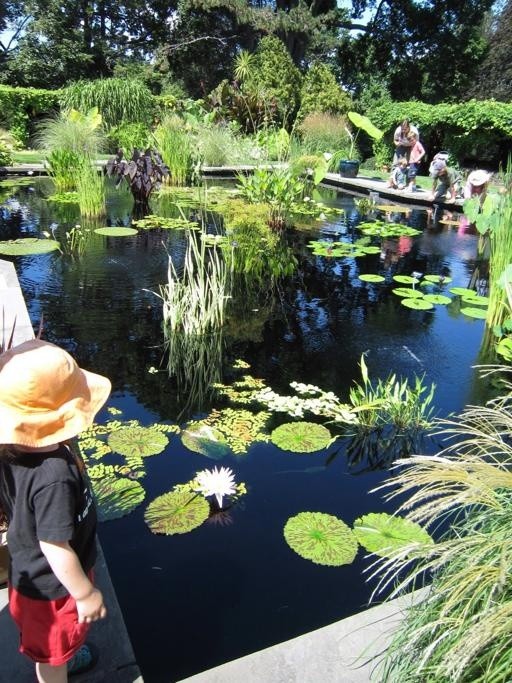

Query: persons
<box><xmin>392</xmin><ymin>119</ymin><xmax>420</xmax><ymax>160</ymax></box>
<box><xmin>464</xmin><ymin>168</ymin><xmax>494</xmax><ymax>200</ymax></box>
<box><xmin>1</xmin><ymin>339</ymin><xmax>112</xmax><ymax>683</ymax></box>
<box><xmin>387</xmin><ymin>156</ymin><xmax>407</xmax><ymax>190</ymax></box>
<box><xmin>430</xmin><ymin>158</ymin><xmax>466</xmax><ymax>202</ymax></box>
<box><xmin>405</xmin><ymin>132</ymin><xmax>425</xmax><ymax>193</ymax></box>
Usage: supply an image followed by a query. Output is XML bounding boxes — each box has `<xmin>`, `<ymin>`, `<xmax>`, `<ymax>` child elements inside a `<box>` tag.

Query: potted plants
<box><xmin>328</xmin><ymin>149</ymin><xmax>359</xmax><ymax>178</ymax></box>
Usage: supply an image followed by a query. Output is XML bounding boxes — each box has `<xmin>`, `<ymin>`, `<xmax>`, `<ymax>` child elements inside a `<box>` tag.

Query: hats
<box><xmin>428</xmin><ymin>160</ymin><xmax>446</xmax><ymax>179</ymax></box>
<box><xmin>469</xmin><ymin>170</ymin><xmax>494</xmax><ymax>186</ymax></box>
<box><xmin>0</xmin><ymin>339</ymin><xmax>113</xmax><ymax>449</ymax></box>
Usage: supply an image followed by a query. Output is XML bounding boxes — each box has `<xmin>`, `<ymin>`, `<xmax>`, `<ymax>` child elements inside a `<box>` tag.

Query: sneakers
<box><xmin>68</xmin><ymin>644</ymin><xmax>98</xmax><ymax>677</ymax></box>
